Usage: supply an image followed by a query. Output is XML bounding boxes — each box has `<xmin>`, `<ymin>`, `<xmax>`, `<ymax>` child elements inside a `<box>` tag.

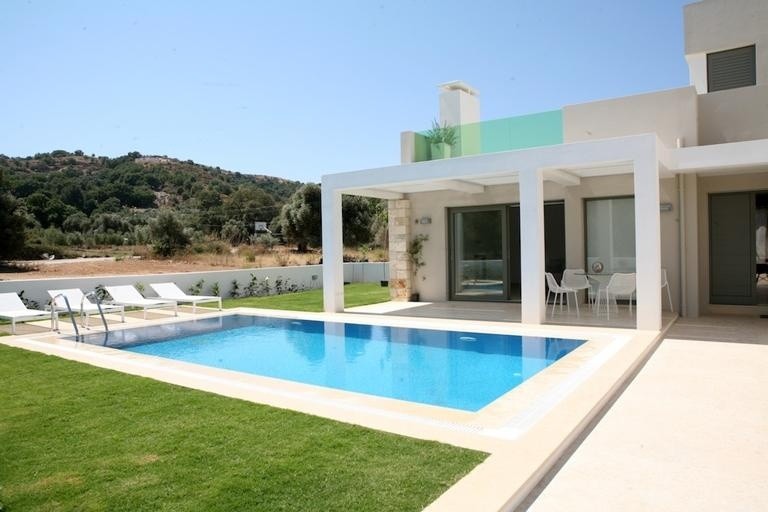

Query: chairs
<box><xmin>0</xmin><ymin>292</ymin><xmax>57</xmax><ymax>334</ymax></box>
<box><xmin>151</xmin><ymin>282</ymin><xmax>221</xmax><ymax>315</ymax></box>
<box><xmin>105</xmin><ymin>286</ymin><xmax>177</xmax><ymax>319</ymax></box>
<box><xmin>560</xmin><ymin>269</ymin><xmax>595</xmax><ymax>314</ymax></box>
<box><xmin>545</xmin><ymin>272</ymin><xmax>580</xmax><ymax>319</ymax></box>
<box><xmin>48</xmin><ymin>289</ymin><xmax>124</xmax><ymax>329</ymax></box>
<box><xmin>597</xmin><ymin>273</ymin><xmax>636</xmax><ymax>319</ymax></box>
<box><xmin>661</xmin><ymin>269</ymin><xmax>674</xmax><ymax>314</ymax></box>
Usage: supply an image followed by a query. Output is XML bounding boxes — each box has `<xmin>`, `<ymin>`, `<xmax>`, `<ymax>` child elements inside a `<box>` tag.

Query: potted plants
<box><xmin>425</xmin><ymin>119</ymin><xmax>456</xmax><ymax>159</ymax></box>
<box><xmin>407</xmin><ymin>234</ymin><xmax>429</xmax><ymax>302</ymax></box>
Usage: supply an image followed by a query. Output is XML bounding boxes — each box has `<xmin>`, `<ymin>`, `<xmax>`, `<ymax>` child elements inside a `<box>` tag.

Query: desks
<box><xmin>575</xmin><ymin>273</ymin><xmax>619</xmax><ymax>313</ymax></box>
